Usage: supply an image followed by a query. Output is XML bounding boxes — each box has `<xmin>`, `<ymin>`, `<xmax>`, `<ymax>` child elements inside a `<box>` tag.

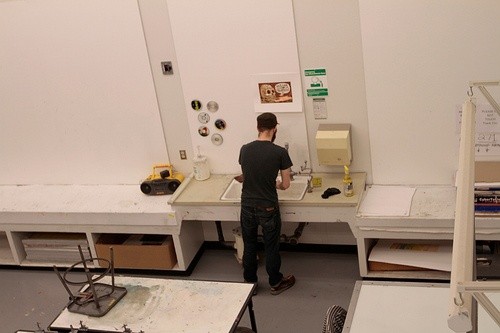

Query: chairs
<box><xmin>323</xmin><ymin>305</ymin><xmax>347</xmax><ymax>333</ymax></box>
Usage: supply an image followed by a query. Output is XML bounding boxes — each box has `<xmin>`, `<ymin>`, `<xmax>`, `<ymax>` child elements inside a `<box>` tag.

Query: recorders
<box><xmin>140</xmin><ymin>164</ymin><xmax>185</xmax><ymax>196</ymax></box>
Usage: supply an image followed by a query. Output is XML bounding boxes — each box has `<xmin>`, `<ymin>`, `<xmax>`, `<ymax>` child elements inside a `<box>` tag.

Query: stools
<box><xmin>51</xmin><ymin>245</ymin><xmax>128</xmax><ymax>318</ymax></box>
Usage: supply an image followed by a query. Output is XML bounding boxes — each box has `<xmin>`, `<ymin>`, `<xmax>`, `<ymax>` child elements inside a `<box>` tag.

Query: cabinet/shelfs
<box><xmin>353</xmin><ymin>185</ymin><xmax>500</xmax><ymax>283</ymax></box>
<box><xmin>0</xmin><ymin>184</ymin><xmax>205</xmax><ymax>277</ymax></box>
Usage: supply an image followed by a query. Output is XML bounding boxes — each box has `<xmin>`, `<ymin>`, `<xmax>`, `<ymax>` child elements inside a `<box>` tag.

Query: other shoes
<box><xmin>270</xmin><ymin>274</ymin><xmax>296</xmax><ymax>295</ymax></box>
<box><xmin>244</xmin><ymin>280</ymin><xmax>257</xmax><ymax>295</ymax></box>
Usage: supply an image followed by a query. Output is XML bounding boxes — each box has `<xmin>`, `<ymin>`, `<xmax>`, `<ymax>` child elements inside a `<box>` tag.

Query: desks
<box><xmin>341</xmin><ymin>280</ymin><xmax>500</xmax><ymax>333</ymax></box>
<box><xmin>47</xmin><ymin>273</ymin><xmax>258</xmax><ymax>333</ymax></box>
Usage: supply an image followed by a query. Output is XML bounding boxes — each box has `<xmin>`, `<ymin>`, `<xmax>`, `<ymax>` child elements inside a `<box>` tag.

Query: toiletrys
<box><xmin>192</xmin><ymin>145</ymin><xmax>210</xmax><ymax>180</ymax></box>
<box><xmin>344</xmin><ymin>165</ymin><xmax>353</xmax><ymax>197</ymax></box>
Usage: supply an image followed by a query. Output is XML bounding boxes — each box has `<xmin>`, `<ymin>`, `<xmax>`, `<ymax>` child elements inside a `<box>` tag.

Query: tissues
<box><xmin>315</xmin><ymin>123</ymin><xmax>353</xmax><ymax>166</ymax></box>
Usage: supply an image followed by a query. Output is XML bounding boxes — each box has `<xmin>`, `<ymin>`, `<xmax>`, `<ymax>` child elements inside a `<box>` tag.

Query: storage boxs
<box><xmin>94</xmin><ymin>234</ymin><xmax>176</xmax><ymax>270</ymax></box>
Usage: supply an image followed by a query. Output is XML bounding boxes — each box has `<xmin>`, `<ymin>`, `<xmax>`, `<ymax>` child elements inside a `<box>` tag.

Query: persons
<box><xmin>238</xmin><ymin>112</ymin><xmax>295</xmax><ymax>296</ymax></box>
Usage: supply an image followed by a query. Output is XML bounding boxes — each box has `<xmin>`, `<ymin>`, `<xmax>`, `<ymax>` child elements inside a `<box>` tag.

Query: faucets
<box><xmin>285</xmin><ymin>142</ymin><xmax>297</xmax><ymax>181</ymax></box>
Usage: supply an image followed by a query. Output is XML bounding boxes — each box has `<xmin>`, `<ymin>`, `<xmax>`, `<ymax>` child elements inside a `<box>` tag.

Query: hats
<box><xmin>257</xmin><ymin>112</ymin><xmax>280</xmax><ymax>127</ymax></box>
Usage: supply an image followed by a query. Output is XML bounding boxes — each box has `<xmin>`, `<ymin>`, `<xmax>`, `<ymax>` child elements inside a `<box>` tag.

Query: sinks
<box><xmin>221</xmin><ymin>176</ymin><xmax>311</xmax><ymax>200</ymax></box>
